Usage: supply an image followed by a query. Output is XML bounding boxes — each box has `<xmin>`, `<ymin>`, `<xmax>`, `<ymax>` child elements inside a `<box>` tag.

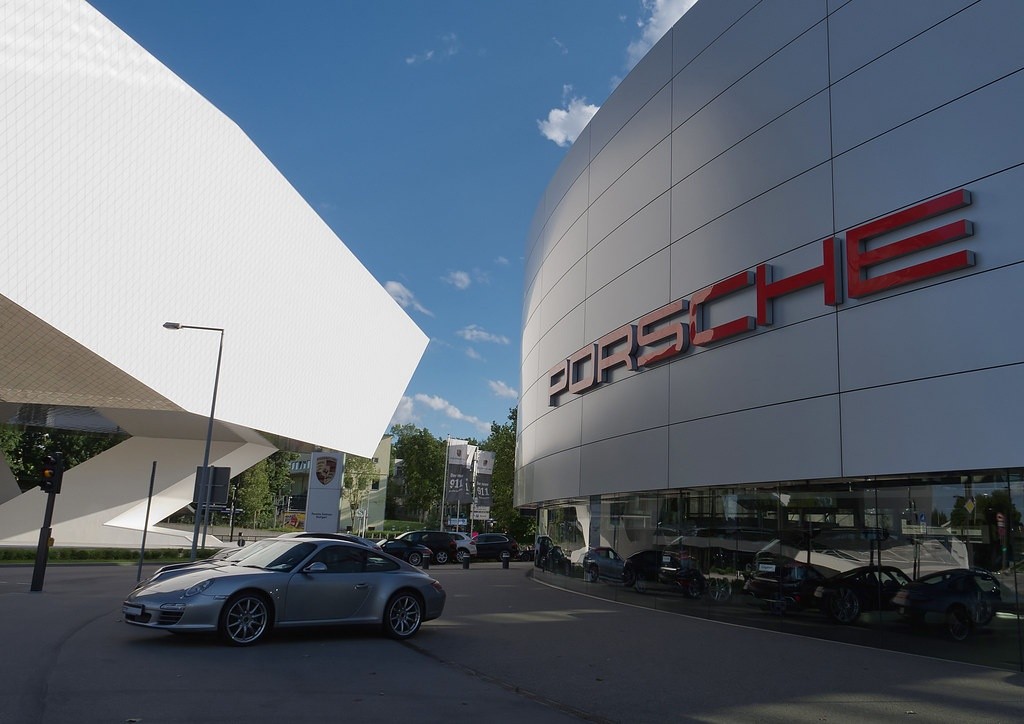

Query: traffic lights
<box><xmin>41</xmin><ymin>455</ymin><xmax>56</xmax><ymax>489</ymax></box>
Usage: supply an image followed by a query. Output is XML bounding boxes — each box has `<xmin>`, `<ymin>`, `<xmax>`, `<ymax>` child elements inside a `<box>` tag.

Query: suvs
<box><xmin>423</xmin><ymin>532</ymin><xmax>477</xmax><ymax>563</ymax></box>
<box><xmin>395</xmin><ymin>531</ymin><xmax>457</xmax><ymax>565</ymax></box>
<box><xmin>472</xmin><ymin>533</ymin><xmax>519</xmax><ymax>562</ymax></box>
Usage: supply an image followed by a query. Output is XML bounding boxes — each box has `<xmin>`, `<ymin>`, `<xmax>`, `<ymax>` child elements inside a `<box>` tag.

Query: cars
<box><xmin>122</xmin><ymin>532</ymin><xmax>446</xmax><ymax>647</ymax></box>
<box><xmin>742</xmin><ymin>532</ymin><xmax>1002</xmax><ymax>627</ymax></box>
<box><xmin>582</xmin><ymin>547</ymin><xmax>625</xmax><ymax>582</ymax></box>
<box><xmin>535</xmin><ymin>534</ymin><xmax>573</xmax><ymax>576</ymax></box>
<box><xmin>623</xmin><ymin>550</ymin><xmax>705</xmax><ymax>599</ymax></box>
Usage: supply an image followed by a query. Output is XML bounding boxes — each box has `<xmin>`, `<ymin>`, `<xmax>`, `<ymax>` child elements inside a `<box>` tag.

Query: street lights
<box><xmin>163</xmin><ymin>323</ymin><xmax>223</xmax><ymax>562</ymax></box>
